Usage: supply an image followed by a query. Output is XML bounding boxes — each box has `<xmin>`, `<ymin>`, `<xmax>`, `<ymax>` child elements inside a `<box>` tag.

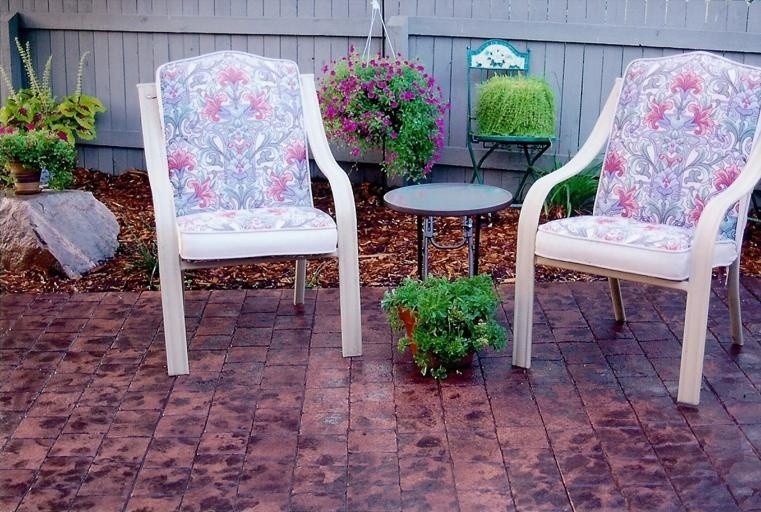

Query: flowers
<box><xmin>314</xmin><ymin>42</ymin><xmax>455</xmax><ymax>183</ymax></box>
<box><xmin>1</xmin><ymin>37</ymin><xmax>109</xmax><ymax>148</ymax></box>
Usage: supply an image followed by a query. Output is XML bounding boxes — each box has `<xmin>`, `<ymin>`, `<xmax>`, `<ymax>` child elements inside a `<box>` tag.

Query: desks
<box><xmin>383</xmin><ymin>181</ymin><xmax>513</xmax><ymax>290</ymax></box>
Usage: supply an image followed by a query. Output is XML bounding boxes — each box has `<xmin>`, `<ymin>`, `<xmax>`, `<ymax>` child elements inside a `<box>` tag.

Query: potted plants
<box><xmin>1</xmin><ymin>124</ymin><xmax>74</xmax><ymax>194</ymax></box>
<box><xmin>377</xmin><ymin>272</ymin><xmax>510</xmax><ymax>385</ymax></box>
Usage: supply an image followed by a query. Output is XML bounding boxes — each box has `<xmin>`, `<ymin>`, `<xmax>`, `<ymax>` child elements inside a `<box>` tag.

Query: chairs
<box><xmin>463</xmin><ymin>39</ymin><xmax>552</xmax><ymax>209</ymax></box>
<box><xmin>511</xmin><ymin>48</ymin><xmax>761</xmax><ymax>407</ymax></box>
<box><xmin>133</xmin><ymin>48</ymin><xmax>364</xmax><ymax>376</ymax></box>
